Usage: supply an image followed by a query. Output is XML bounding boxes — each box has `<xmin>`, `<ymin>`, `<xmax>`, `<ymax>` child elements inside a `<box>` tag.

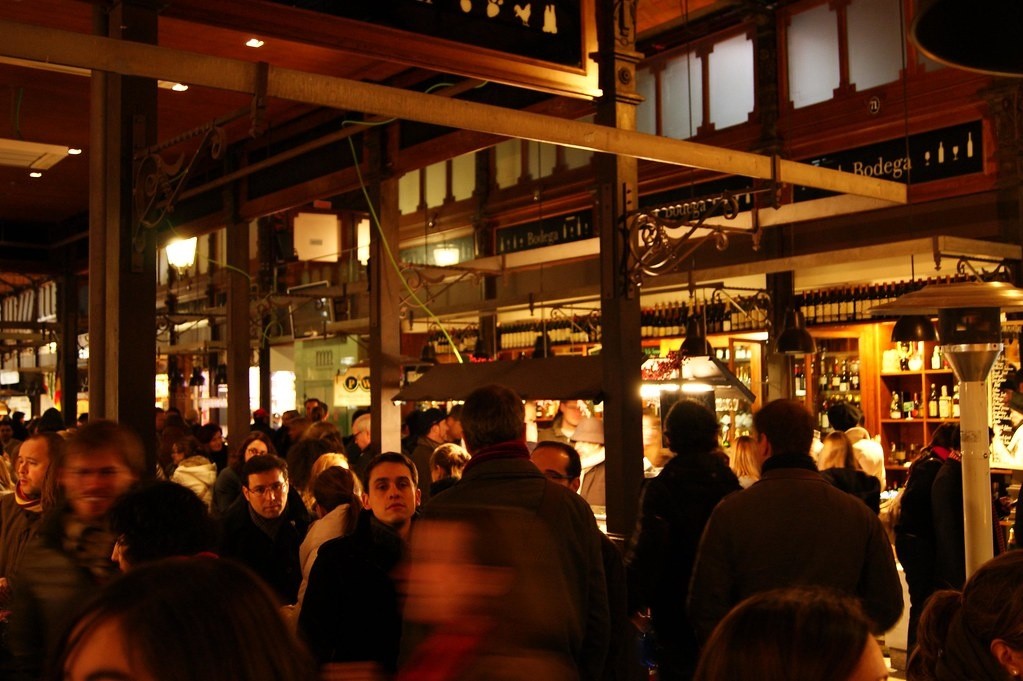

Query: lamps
<box><xmin>415</xmin><ymin>208</ymin><xmax>440</xmax><ymax>374</ymax></box>
<box><xmin>773</xmin><ymin>11</ymin><xmax>817</xmax><ymax>358</ymax></box>
<box><xmin>888</xmin><ymin>1</ymin><xmax>938</xmax><ymax>343</ymax></box>
<box><xmin>675</xmin><ymin>2</ymin><xmax>718</xmax><ymax>362</ymax></box>
<box><xmin>189</xmin><ymin>239</ymin><xmax>205</xmax><ymax>387</ymax></box>
<box><xmin>213</xmin><ymin>298</ymin><xmax>227</xmax><ymax>385</ymax></box>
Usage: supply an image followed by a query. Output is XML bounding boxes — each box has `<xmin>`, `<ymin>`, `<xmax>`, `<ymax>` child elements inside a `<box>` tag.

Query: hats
<box><xmin>253</xmin><ymin>409</ymin><xmax>271</xmax><ymax>418</ymax></box>
<box><xmin>569</xmin><ymin>417</ymin><xmax>604</xmax><ymax>444</ymax></box>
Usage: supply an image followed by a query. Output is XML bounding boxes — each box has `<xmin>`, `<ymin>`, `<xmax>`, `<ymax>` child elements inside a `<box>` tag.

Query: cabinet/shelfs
<box><xmin>429</xmin><ymin>316</ymin><xmax>1023</xmax><ymax>547</ymax></box>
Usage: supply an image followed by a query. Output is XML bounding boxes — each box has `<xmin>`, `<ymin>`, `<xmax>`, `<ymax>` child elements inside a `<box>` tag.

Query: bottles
<box><xmin>794</xmin><ymin>355</ymin><xmax>862</xmax><ymax>428</ymax></box>
<box><xmin>886</xmin><ymin>478</ymin><xmax>898</xmax><ymax>492</ymax></box>
<box><xmin>640</xmin><ymin>293</ymin><xmax>766</xmax><ymax>338</ymax></box>
<box><xmin>712</xmin><ymin>350</ymin><xmax>750</xmax><ymax>411</ymax></box>
<box><xmin>425</xmin><ymin>309</ymin><xmax>603</xmax><ymax>353</ymax></box>
<box><xmin>793</xmin><ymin>272</ymin><xmax>970</xmax><ymax>326</ymax></box>
<box><xmin>882</xmin><ymin>340</ymin><xmax>962</xmax><ymax>420</ymax></box>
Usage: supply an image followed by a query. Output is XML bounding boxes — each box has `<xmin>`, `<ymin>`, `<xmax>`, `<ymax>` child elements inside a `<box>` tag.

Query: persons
<box><xmin>0</xmin><ymin>387</ymin><xmax>1023</xmax><ymax>681</ymax></box>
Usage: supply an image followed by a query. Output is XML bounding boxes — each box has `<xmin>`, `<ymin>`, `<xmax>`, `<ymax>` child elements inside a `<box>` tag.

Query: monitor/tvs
<box><xmin>287</xmin><ymin>280</ymin><xmax>337</xmax><ymax>340</ymax></box>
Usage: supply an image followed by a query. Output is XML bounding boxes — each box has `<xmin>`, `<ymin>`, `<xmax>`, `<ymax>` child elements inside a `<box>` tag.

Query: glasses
<box><xmin>352</xmin><ymin>430</ymin><xmax>364</xmax><ymax>440</ymax></box>
<box><xmin>247</xmin><ymin>479</ymin><xmax>287</xmax><ymax>495</ymax></box>
<box><xmin>247</xmin><ymin>448</ymin><xmax>266</xmax><ymax>456</ymax></box>
<box><xmin>543</xmin><ymin>472</ymin><xmax>574</xmax><ymax>484</ymax></box>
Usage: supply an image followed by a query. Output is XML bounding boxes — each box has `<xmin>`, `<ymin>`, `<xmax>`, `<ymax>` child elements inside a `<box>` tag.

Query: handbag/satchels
<box><xmin>878</xmin><ymin>471</ymin><xmax>911</xmax><ymax>546</ymax></box>
